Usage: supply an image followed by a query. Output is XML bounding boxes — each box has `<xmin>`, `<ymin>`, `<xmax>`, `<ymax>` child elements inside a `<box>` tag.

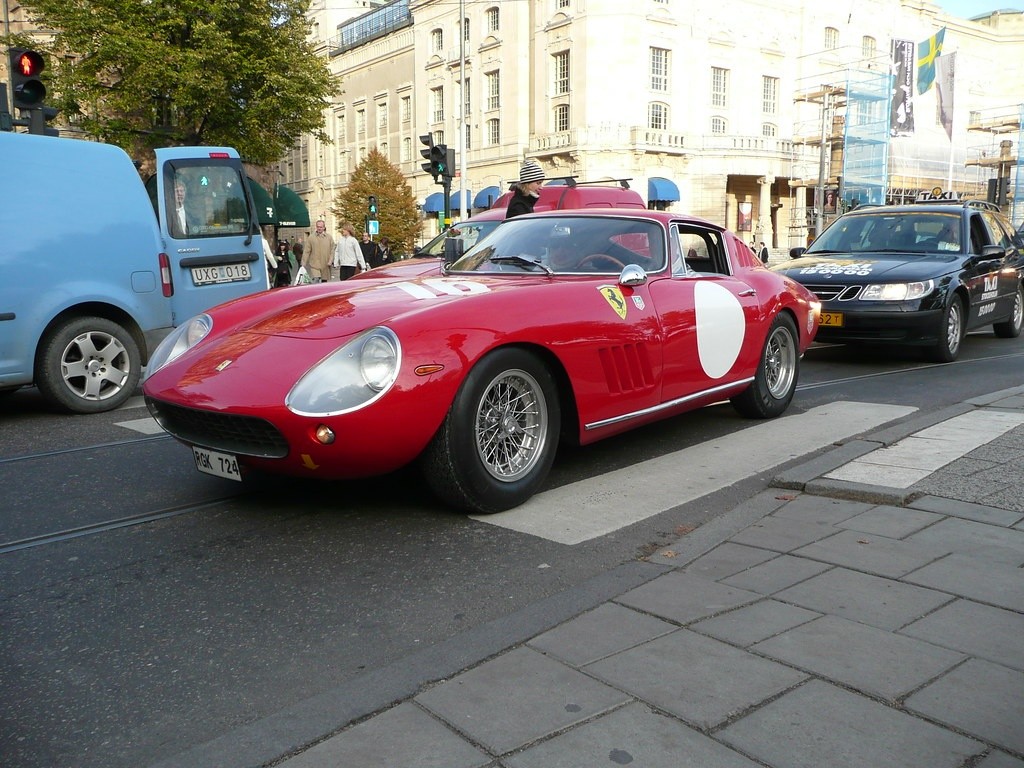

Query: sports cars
<box><xmin>141</xmin><ymin>207</ymin><xmax>822</xmax><ymax>515</ymax></box>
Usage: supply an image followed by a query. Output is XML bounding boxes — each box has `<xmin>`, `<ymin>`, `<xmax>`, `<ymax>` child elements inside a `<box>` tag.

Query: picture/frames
<box><xmin>738</xmin><ymin>202</ymin><xmax>753</xmax><ymax>232</ymax></box>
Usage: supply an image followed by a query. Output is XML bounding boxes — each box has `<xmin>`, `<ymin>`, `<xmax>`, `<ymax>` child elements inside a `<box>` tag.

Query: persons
<box><xmin>758</xmin><ymin>241</ymin><xmax>769</xmax><ymax>268</ymax></box>
<box><xmin>824</xmin><ymin>193</ymin><xmax>835</xmax><ymax>212</ymax></box>
<box><xmin>379</xmin><ymin>238</ymin><xmax>395</xmax><ymax>263</ymax></box>
<box><xmin>505</xmin><ymin>165</ymin><xmax>545</xmax><ymax>219</ymax></box>
<box><xmin>165</xmin><ymin>179</ymin><xmax>197</xmax><ymax>239</ymax></box>
<box><xmin>334</xmin><ymin>223</ymin><xmax>365</xmax><ymax>281</ymax></box>
<box><xmin>748</xmin><ymin>241</ymin><xmax>756</xmax><ymax>254</ymax></box>
<box><xmin>267</xmin><ymin>236</ymin><xmax>304</xmax><ymax>288</ymax></box>
<box><xmin>533</xmin><ymin>237</ymin><xmax>577</xmax><ymax>270</ymax></box>
<box><xmin>300</xmin><ymin>220</ymin><xmax>336</xmax><ymax>283</ymax></box>
<box><xmin>358</xmin><ymin>232</ymin><xmax>379</xmax><ymax>269</ymax></box>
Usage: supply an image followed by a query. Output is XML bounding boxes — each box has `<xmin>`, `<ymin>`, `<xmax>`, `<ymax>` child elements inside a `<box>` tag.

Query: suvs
<box><xmin>766</xmin><ymin>187</ymin><xmax>1024</xmax><ymax>364</ymax></box>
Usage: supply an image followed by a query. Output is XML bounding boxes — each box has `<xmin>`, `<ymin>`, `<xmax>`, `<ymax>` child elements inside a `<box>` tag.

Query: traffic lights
<box><xmin>31</xmin><ymin>107</ymin><xmax>60</xmax><ymax>137</ymax></box>
<box><xmin>432</xmin><ymin>144</ymin><xmax>449</xmax><ymax>174</ymax></box>
<box><xmin>9</xmin><ymin>48</ymin><xmax>46</xmax><ymax>109</ymax></box>
<box><xmin>998</xmin><ymin>177</ymin><xmax>1011</xmax><ymax>206</ymax></box>
<box><xmin>419</xmin><ymin>132</ymin><xmax>434</xmax><ymax>173</ymax></box>
<box><xmin>198</xmin><ymin>175</ymin><xmax>211</xmax><ymax>187</ymax></box>
<box><xmin>369</xmin><ymin>196</ymin><xmax>378</xmax><ymax>213</ymax></box>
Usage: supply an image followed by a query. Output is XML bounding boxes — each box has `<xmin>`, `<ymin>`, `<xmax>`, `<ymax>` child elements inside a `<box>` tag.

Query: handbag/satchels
<box><xmin>294</xmin><ymin>266</ymin><xmax>310</xmax><ymax>286</ymax></box>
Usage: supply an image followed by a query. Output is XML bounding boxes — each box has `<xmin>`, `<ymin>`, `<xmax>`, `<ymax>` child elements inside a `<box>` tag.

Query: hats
<box><xmin>825</xmin><ymin>190</ymin><xmax>833</xmax><ymax>198</ymax></box>
<box><xmin>520</xmin><ymin>160</ymin><xmax>545</xmax><ymax>183</ymax></box>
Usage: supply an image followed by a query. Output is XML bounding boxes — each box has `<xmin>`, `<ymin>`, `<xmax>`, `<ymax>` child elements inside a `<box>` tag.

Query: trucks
<box><xmin>0</xmin><ymin>131</ymin><xmax>268</xmax><ymax>415</ymax></box>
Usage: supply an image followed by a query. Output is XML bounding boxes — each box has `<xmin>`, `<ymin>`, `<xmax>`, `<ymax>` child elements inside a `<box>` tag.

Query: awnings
<box><xmin>648</xmin><ymin>176</ymin><xmax>681</xmax><ymax>203</ymax></box>
<box><xmin>145</xmin><ymin>171</ymin><xmax>195</xmax><ymax>210</ymax></box>
<box><xmin>473</xmin><ymin>186</ymin><xmax>499</xmax><ymax>209</ymax></box>
<box><xmin>423</xmin><ymin>192</ymin><xmax>444</xmax><ymax>213</ymax></box>
<box><xmin>224</xmin><ymin>174</ymin><xmax>279</xmax><ymax>227</ymax></box>
<box><xmin>274</xmin><ymin>185</ymin><xmax>311</xmax><ymax>228</ymax></box>
<box><xmin>450</xmin><ymin>189</ymin><xmax>472</xmax><ymax>210</ymax></box>
<box><xmin>544</xmin><ymin>177</ymin><xmax>569</xmax><ymax>185</ymax></box>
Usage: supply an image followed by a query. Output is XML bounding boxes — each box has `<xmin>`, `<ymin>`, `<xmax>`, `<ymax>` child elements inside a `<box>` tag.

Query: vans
<box><xmin>346</xmin><ymin>175</ymin><xmax>652</xmax><ymax>280</ymax></box>
